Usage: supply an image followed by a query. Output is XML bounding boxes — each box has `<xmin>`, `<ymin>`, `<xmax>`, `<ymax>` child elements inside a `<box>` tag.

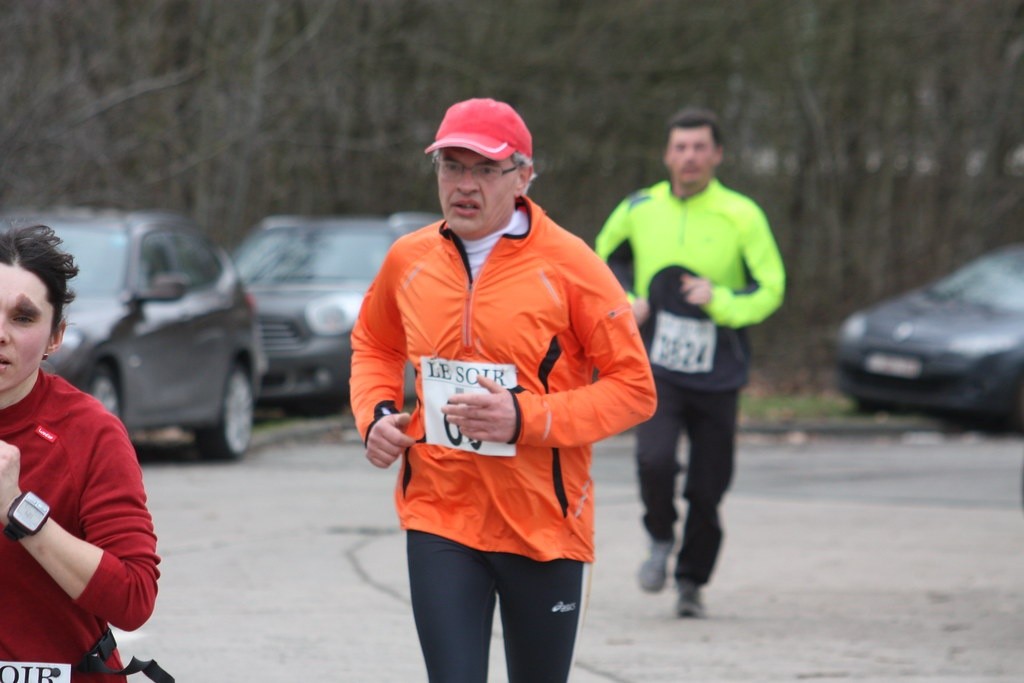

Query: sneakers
<box><xmin>638</xmin><ymin>540</ymin><xmax>675</xmax><ymax>593</ymax></box>
<box><xmin>676</xmin><ymin>584</ymin><xmax>704</xmax><ymax>616</ymax></box>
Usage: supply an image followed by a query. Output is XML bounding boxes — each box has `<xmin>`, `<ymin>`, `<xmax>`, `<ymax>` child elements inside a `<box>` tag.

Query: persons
<box><xmin>594</xmin><ymin>109</ymin><xmax>787</xmax><ymax>615</ymax></box>
<box><xmin>0</xmin><ymin>225</ymin><xmax>176</xmax><ymax>683</ymax></box>
<box><xmin>349</xmin><ymin>99</ymin><xmax>659</xmax><ymax>683</ymax></box>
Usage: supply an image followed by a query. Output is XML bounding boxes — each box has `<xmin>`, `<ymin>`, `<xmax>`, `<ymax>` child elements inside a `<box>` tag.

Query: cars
<box><xmin>235</xmin><ymin>211</ymin><xmax>445</xmax><ymax>418</ymax></box>
<box><xmin>832</xmin><ymin>245</ymin><xmax>1024</xmax><ymax>432</ymax></box>
<box><xmin>0</xmin><ymin>208</ymin><xmax>270</xmax><ymax>459</ymax></box>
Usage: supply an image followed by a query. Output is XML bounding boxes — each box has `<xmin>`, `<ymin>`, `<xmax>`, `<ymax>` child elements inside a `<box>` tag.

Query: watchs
<box><xmin>3</xmin><ymin>490</ymin><xmax>51</xmax><ymax>541</ymax></box>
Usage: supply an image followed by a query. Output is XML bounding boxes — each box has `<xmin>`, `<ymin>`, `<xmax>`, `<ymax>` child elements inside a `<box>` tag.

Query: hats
<box><xmin>425</xmin><ymin>98</ymin><xmax>532</xmax><ymax>162</ymax></box>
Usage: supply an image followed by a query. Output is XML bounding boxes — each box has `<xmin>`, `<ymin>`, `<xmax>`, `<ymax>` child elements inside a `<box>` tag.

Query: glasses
<box><xmin>431</xmin><ymin>151</ymin><xmax>522</xmax><ymax>185</ymax></box>
